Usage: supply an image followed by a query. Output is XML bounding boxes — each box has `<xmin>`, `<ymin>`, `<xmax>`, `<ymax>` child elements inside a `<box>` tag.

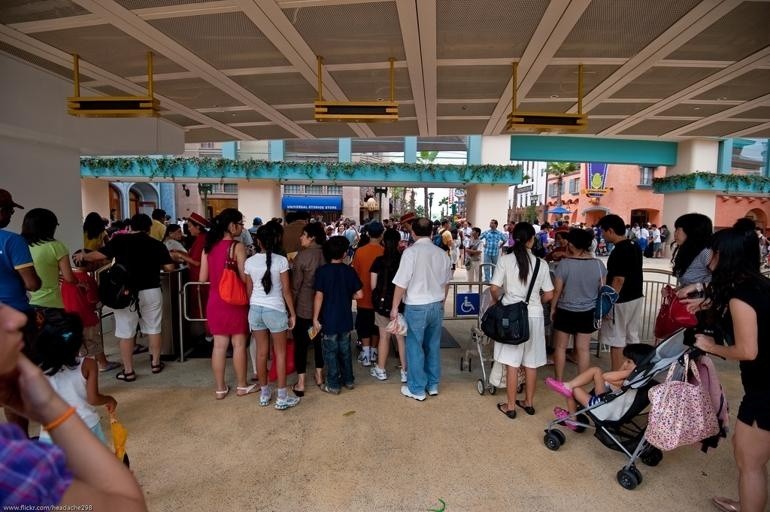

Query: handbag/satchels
<box><xmin>654</xmin><ymin>301</ymin><xmax>698</xmax><ymax>338</ymax></box>
<box><xmin>489</xmin><ymin>361</ymin><xmax>525</xmax><ymax>389</ymax></box>
<box><xmin>219</xmin><ymin>258</ymin><xmax>249</xmax><ymax>305</ymax></box>
<box><xmin>481</xmin><ymin>300</ymin><xmax>529</xmax><ymax>345</ymax></box>
<box><xmin>645</xmin><ymin>381</ymin><xmax>720</xmax><ymax>453</ymax></box>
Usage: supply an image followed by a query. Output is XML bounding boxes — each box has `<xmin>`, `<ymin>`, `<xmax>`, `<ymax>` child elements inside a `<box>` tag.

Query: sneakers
<box><xmin>357</xmin><ymin>349</ymin><xmax>388</xmax><ymax>381</ymax></box>
<box><xmin>320</xmin><ymin>379</ymin><xmax>354</xmax><ymax>395</ymax></box>
<box><xmin>257</xmin><ymin>388</ymin><xmax>301</xmax><ymax>411</ymax></box>
<box><xmin>400</xmin><ymin>369</ymin><xmax>439</xmax><ymax>401</ymax></box>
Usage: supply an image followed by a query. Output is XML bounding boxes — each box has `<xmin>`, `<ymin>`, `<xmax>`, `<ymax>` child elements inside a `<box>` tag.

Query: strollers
<box><xmin>596</xmin><ymin>237</ymin><xmax>608</xmax><ymax>256</ymax></box>
<box><xmin>545</xmin><ymin>320</ymin><xmax>729</xmax><ymax>490</ymax></box>
<box><xmin>460</xmin><ymin>289</ymin><xmax>526</xmax><ymax>401</ymax></box>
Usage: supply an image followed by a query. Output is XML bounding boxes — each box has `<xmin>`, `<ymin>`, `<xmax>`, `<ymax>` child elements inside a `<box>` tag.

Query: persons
<box><xmin>682</xmin><ymin>225</ymin><xmax>769</xmax><ymax>510</ymax></box>
<box><xmin>0</xmin><ymin>185</ymin><xmax>770</xmax><ymax>432</ymax></box>
<box><xmin>29</xmin><ymin>309</ymin><xmax>118</xmax><ymax>467</ymax></box>
<box><xmin>0</xmin><ymin>299</ymin><xmax>152</xmax><ymax>512</ymax></box>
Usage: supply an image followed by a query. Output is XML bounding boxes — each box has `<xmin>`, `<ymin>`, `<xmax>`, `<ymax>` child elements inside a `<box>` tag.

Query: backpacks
<box><xmin>372</xmin><ymin>268</ymin><xmax>405</xmax><ymax>316</ymax></box>
<box><xmin>595</xmin><ymin>286</ymin><xmax>620</xmax><ymax>321</ymax></box>
<box><xmin>693</xmin><ymin>356</ymin><xmax>730</xmax><ymax>449</ymax></box>
<box><xmin>101</xmin><ymin>265</ymin><xmax>135</xmax><ymax>309</ymax></box>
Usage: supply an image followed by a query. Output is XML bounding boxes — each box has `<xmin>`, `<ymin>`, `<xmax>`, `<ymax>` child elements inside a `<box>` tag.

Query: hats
<box><xmin>0</xmin><ymin>188</ymin><xmax>24</xmax><ymax>210</ymax></box>
<box><xmin>183</xmin><ymin>212</ymin><xmax>208</xmax><ymax>228</ymax></box>
<box><xmin>399</xmin><ymin>212</ymin><xmax>416</xmax><ymax>225</ymax></box>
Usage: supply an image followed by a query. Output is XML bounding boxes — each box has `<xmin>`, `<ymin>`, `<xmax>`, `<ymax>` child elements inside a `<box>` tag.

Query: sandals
<box><xmin>236</xmin><ymin>383</ymin><xmax>260</xmax><ymax>395</ymax></box>
<box><xmin>116</xmin><ymin>368</ymin><xmax>137</xmax><ymax>382</ymax></box>
<box><xmin>215</xmin><ymin>385</ymin><xmax>230</xmax><ymax>400</ymax></box>
<box><xmin>100</xmin><ymin>361</ymin><xmax>120</xmax><ymax>371</ymax></box>
<box><xmin>151</xmin><ymin>359</ymin><xmax>165</xmax><ymax>374</ymax></box>
<box><xmin>552</xmin><ymin>406</ymin><xmax>577</xmax><ymax>430</ymax></box>
<box><xmin>544</xmin><ymin>376</ymin><xmax>572</xmax><ymax>397</ymax></box>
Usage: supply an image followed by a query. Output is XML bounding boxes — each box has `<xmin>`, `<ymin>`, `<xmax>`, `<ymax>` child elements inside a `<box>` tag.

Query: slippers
<box><xmin>290</xmin><ymin>381</ymin><xmax>306</xmax><ymax>396</ymax></box>
<box><xmin>133</xmin><ymin>342</ymin><xmax>148</xmax><ymax>355</ymax></box>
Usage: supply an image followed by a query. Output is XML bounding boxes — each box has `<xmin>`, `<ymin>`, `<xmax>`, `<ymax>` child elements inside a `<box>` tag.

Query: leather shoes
<box><xmin>497</xmin><ymin>399</ymin><xmax>535</xmax><ymax>418</ymax></box>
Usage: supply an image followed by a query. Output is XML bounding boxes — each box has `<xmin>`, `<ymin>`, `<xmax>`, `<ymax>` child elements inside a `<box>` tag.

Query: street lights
<box><xmin>428</xmin><ymin>192</ymin><xmax>435</xmax><ymax>223</ymax></box>
<box><xmin>198</xmin><ymin>183</ymin><xmax>214</xmax><ymax>220</ymax></box>
<box><xmin>367</xmin><ymin>197</ymin><xmax>376</xmax><ymax>221</ymax></box>
